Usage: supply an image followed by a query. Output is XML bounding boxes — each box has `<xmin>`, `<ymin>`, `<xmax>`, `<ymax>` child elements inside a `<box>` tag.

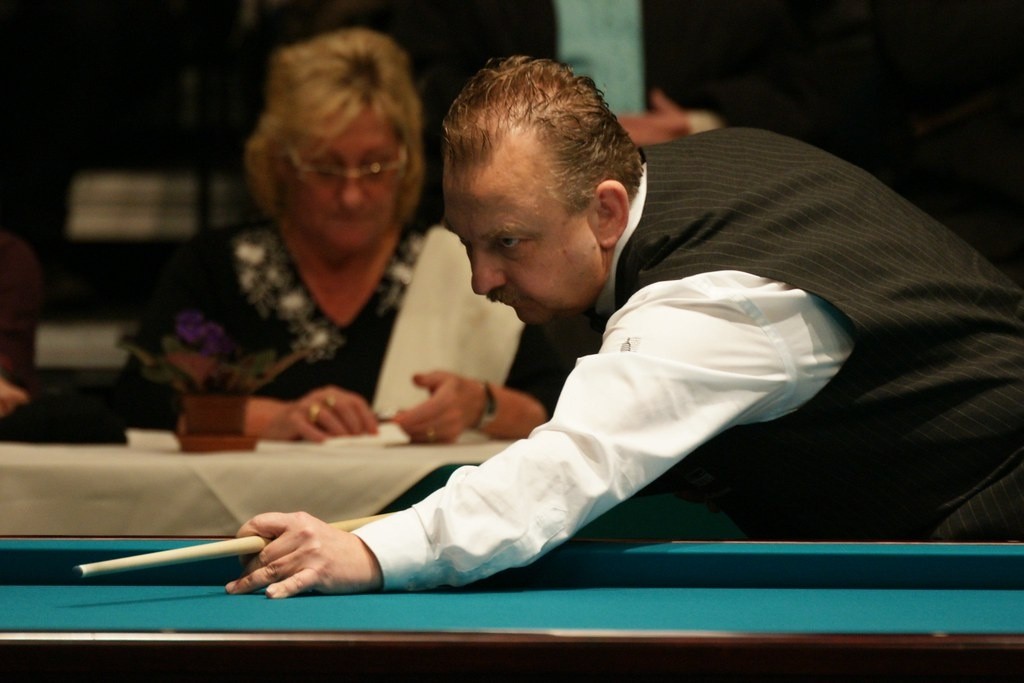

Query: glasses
<box><xmin>289</xmin><ymin>140</ymin><xmax>407</xmax><ymax>185</ymax></box>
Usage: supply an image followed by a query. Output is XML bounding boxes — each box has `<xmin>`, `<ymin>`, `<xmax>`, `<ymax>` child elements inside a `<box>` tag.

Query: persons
<box><xmin>121</xmin><ymin>21</ymin><xmax>604</xmax><ymax>443</ymax></box>
<box><xmin>1</xmin><ymin>232</ymin><xmax>119</xmax><ymax>445</ymax></box>
<box><xmin>217</xmin><ymin>54</ymin><xmax>1024</xmax><ymax>602</ymax></box>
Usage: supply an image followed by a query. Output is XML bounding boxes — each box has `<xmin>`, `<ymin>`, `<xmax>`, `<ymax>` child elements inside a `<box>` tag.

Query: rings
<box><xmin>325</xmin><ymin>392</ymin><xmax>338</xmax><ymax>407</ymax></box>
<box><xmin>426</xmin><ymin>430</ymin><xmax>437</xmax><ymax>441</ymax></box>
<box><xmin>305</xmin><ymin>402</ymin><xmax>321</xmax><ymax>425</ymax></box>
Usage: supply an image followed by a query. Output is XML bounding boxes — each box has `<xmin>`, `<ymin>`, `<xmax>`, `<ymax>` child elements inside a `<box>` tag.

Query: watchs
<box><xmin>471</xmin><ymin>378</ymin><xmax>498</xmax><ymax>429</ymax></box>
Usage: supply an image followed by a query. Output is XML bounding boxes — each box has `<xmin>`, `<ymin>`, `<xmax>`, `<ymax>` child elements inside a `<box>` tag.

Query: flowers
<box><xmin>122</xmin><ymin>313</ymin><xmax>316</xmax><ymax>389</ymax></box>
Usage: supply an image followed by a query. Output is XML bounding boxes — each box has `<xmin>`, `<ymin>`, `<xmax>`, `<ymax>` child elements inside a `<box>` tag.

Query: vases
<box><xmin>177</xmin><ymin>396</ymin><xmax>260</xmax><ymax>450</ymax></box>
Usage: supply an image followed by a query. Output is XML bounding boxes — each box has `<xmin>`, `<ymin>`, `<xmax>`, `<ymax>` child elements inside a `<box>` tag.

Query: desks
<box><xmin>0</xmin><ymin>530</ymin><xmax>1024</xmax><ymax>683</ymax></box>
<box><xmin>0</xmin><ymin>426</ymin><xmax>529</xmax><ymax>536</ymax></box>
